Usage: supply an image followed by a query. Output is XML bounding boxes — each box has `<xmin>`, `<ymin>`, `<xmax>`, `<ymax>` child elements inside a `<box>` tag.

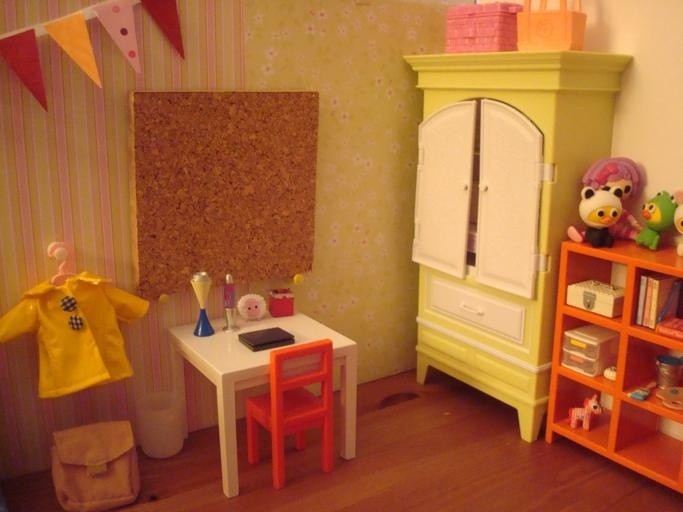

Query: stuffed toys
<box><xmin>666</xmin><ymin>204</ymin><xmax>683</xmax><ymax>258</ymax></box>
<box><xmin>575</xmin><ymin>185</ymin><xmax>623</xmax><ymax>248</ymax></box>
<box><xmin>559</xmin><ymin>151</ymin><xmax>659</xmax><ymax>250</ymax></box>
<box><xmin>235</xmin><ymin>293</ymin><xmax>266</xmax><ymax>322</ymax></box>
<box><xmin>567</xmin><ymin>393</ymin><xmax>602</xmax><ymax>431</ymax></box>
<box><xmin>632</xmin><ymin>187</ymin><xmax>677</xmax><ymax>250</ymax></box>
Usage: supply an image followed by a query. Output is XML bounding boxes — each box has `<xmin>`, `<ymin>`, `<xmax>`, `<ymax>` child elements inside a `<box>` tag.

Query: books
<box><xmin>648</xmin><ymin>278</ymin><xmax>681</xmax><ymax>330</ymax></box>
<box><xmin>237</xmin><ymin>337</ymin><xmax>295</xmax><ymax>352</ymax></box>
<box><xmin>238</xmin><ymin>327</ymin><xmax>295</xmax><ymax>346</ymax></box>
<box><xmin>634</xmin><ymin>272</ymin><xmax>664</xmax><ymax>325</ymax></box>
<box><xmin>640</xmin><ymin>274</ymin><xmax>674</xmax><ymax>328</ymax></box>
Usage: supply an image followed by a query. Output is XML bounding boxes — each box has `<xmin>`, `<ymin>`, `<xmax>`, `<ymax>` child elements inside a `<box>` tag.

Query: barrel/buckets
<box><xmin>655</xmin><ymin>354</ymin><xmax>683</xmax><ymax>393</ymax></box>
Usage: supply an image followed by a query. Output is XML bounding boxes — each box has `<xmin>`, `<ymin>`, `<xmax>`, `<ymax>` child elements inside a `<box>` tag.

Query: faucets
<box><xmin>136</xmin><ymin>392</ymin><xmax>183</xmax><ymax>458</ymax></box>
